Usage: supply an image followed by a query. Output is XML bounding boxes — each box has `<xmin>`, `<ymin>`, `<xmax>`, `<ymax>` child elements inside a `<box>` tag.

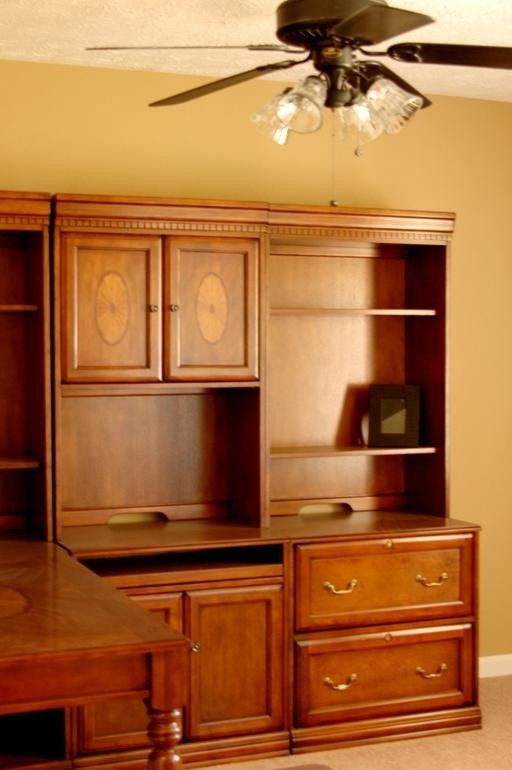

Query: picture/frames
<box><xmin>368</xmin><ymin>384</ymin><xmax>420</xmax><ymax>447</ymax></box>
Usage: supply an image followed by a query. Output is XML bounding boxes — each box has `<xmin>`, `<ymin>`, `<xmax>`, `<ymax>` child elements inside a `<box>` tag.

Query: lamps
<box><xmin>249</xmin><ymin>46</ymin><xmax>422</xmax><ymax>148</ymax></box>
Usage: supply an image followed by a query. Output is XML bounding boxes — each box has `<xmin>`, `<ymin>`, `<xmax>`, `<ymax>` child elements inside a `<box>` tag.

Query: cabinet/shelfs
<box><xmin>0</xmin><ymin>191</ymin><xmax>52</xmax><ymax>769</ymax></box>
<box><xmin>71</xmin><ymin>564</ymin><xmax>289</xmax><ymax>770</ymax></box>
<box><xmin>265</xmin><ymin>203</ymin><xmax>456</xmax><ymax>540</ymax></box>
<box><xmin>53</xmin><ymin>194</ymin><xmax>269</xmax><ymax>397</ymax></box>
<box><xmin>289</xmin><ymin>534</ymin><xmax>478</xmax><ymax>729</ymax></box>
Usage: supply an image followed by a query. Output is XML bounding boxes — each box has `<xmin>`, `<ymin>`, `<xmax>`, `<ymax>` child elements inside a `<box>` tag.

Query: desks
<box><xmin>0</xmin><ymin>543</ymin><xmax>194</xmax><ymax>769</ymax></box>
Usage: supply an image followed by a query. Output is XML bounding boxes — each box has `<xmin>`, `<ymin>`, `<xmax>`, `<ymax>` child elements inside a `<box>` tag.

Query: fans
<box><xmin>86</xmin><ymin>1</ymin><xmax>512</xmax><ymax>109</ymax></box>
